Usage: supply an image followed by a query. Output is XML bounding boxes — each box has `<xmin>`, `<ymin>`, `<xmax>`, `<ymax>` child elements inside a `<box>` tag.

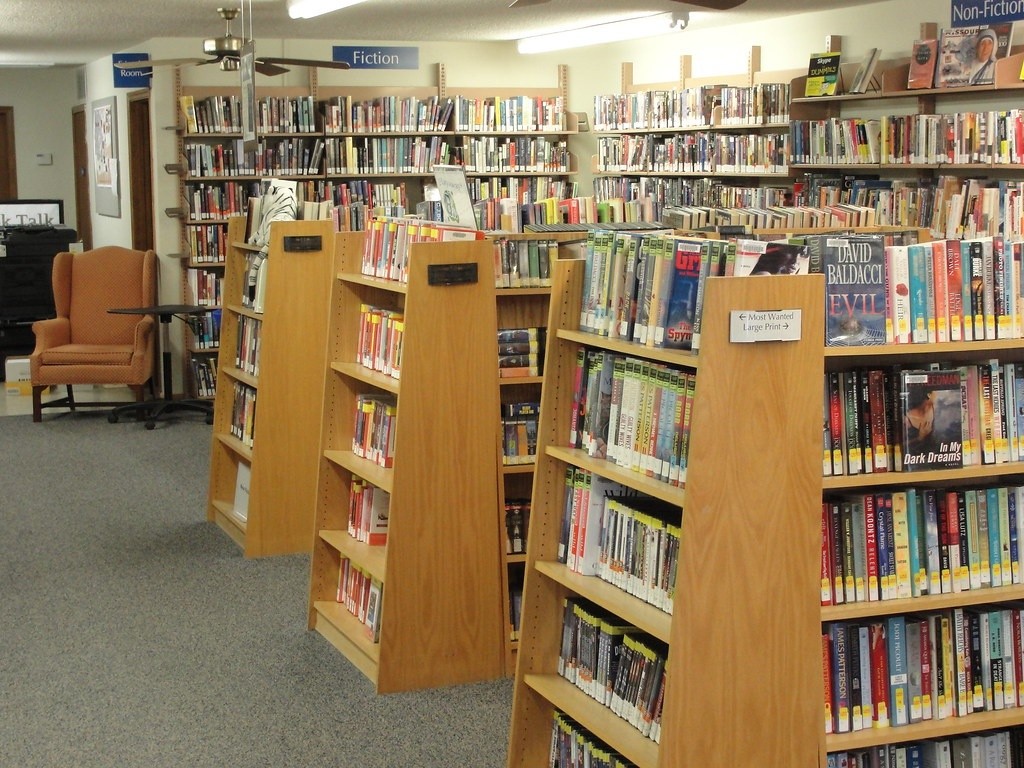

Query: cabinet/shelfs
<box><xmin>156</xmin><ymin>20</ymin><xmax>1023</xmax><ymax>768</ymax></box>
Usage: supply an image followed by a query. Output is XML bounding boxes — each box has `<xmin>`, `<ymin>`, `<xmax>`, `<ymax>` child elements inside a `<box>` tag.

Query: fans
<box><xmin>113</xmin><ymin>9</ymin><xmax>351</xmax><ymax>76</ymax></box>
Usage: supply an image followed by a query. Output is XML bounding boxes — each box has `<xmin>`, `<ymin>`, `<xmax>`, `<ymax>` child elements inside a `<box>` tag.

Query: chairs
<box><xmin>27</xmin><ymin>246</ymin><xmax>155</xmax><ymax>425</ymax></box>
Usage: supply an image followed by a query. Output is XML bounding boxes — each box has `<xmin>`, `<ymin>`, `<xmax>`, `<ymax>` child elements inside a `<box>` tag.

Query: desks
<box><xmin>104</xmin><ymin>304</ymin><xmax>216</xmax><ymax>430</ymax></box>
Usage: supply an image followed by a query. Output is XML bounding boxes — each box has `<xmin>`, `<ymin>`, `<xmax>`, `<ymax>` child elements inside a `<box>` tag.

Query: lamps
<box><xmin>516</xmin><ymin>9</ymin><xmax>690</xmax><ymax>55</ymax></box>
<box><xmin>286</xmin><ymin>1</ymin><xmax>362</xmax><ymax>18</ymax></box>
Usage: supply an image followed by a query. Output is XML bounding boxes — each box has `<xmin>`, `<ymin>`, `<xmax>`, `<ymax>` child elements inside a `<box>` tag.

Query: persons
<box><xmin>749</xmin><ymin>243</ymin><xmax>810</xmax><ymax>276</ymax></box>
<box><xmin>907</xmin><ymin>388</ymin><xmax>936</xmax><ymax>455</ymax></box>
<box><xmin>970</xmin><ymin>28</ymin><xmax>997</xmax><ymax>85</ymax></box>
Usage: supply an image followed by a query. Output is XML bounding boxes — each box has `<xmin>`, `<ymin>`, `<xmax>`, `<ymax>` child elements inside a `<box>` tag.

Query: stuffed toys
<box><xmin>249</xmin><ymin>185</ymin><xmax>298</xmax><ymax>302</ymax></box>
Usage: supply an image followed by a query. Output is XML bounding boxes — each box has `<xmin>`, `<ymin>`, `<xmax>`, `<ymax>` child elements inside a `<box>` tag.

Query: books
<box><xmin>179</xmin><ymin>12</ymin><xmax>1024</xmax><ymax>768</ymax></box>
